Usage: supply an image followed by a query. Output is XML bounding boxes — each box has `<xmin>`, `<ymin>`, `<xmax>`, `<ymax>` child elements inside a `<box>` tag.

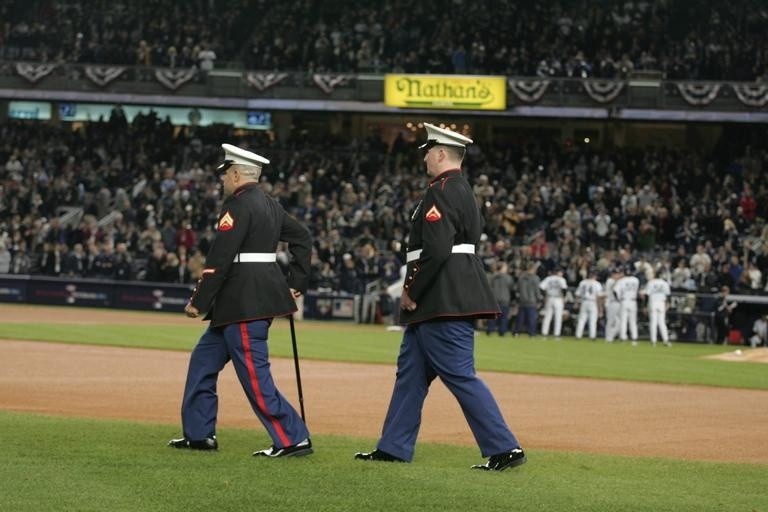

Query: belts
<box><xmin>406</xmin><ymin>243</ymin><xmax>476</xmax><ymax>264</ymax></box>
<box><xmin>233</xmin><ymin>252</ymin><xmax>277</xmax><ymax>264</ymax></box>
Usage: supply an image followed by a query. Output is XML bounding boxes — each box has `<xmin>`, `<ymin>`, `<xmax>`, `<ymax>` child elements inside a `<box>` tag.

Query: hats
<box><xmin>417</xmin><ymin>121</ymin><xmax>474</xmax><ymax>151</ymax></box>
<box><xmin>215</xmin><ymin>143</ymin><xmax>270</xmax><ymax>177</ymax></box>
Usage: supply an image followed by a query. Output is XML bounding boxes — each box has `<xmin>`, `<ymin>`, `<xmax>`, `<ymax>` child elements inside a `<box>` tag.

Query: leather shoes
<box><xmin>252</xmin><ymin>436</ymin><xmax>314</xmax><ymax>459</ymax></box>
<box><xmin>470</xmin><ymin>444</ymin><xmax>528</xmax><ymax>472</ymax></box>
<box><xmin>354</xmin><ymin>447</ymin><xmax>405</xmax><ymax>463</ymax></box>
<box><xmin>167</xmin><ymin>435</ymin><xmax>219</xmax><ymax>452</ymax></box>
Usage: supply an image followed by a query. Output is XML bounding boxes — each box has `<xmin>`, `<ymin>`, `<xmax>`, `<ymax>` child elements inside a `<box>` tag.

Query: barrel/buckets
<box><xmin>729</xmin><ymin>327</ymin><xmax>742</xmax><ymax>345</ymax></box>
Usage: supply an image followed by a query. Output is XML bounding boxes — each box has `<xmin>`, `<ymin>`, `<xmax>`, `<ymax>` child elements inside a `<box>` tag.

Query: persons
<box><xmin>166</xmin><ymin>143</ymin><xmax>314</xmax><ymax>460</ymax></box>
<box><xmin>353</xmin><ymin>125</ymin><xmax>527</xmax><ymax>473</ymax></box>
